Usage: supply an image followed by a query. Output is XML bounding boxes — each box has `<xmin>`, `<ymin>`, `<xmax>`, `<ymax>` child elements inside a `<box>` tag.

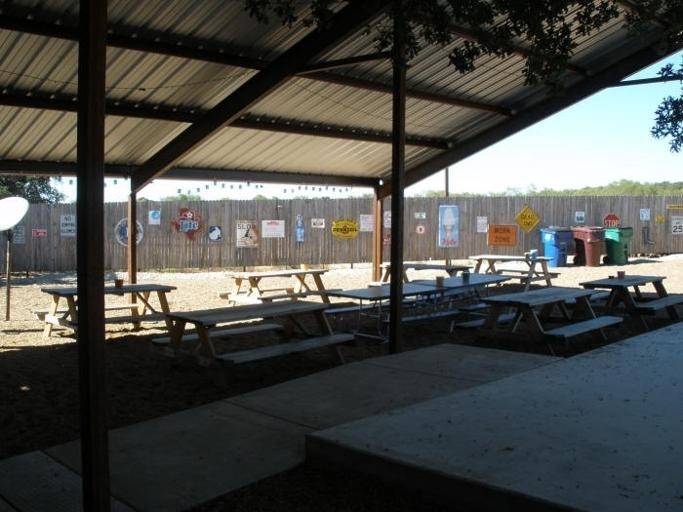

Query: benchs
<box><xmin>32</xmin><ymin>283</ymin><xmax>179</xmax><ymax>339</ymax></box>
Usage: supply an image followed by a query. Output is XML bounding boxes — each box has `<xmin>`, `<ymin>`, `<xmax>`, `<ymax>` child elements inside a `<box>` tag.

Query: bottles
<box><xmin>462</xmin><ymin>270</ymin><xmax>470</xmax><ymax>281</ymax></box>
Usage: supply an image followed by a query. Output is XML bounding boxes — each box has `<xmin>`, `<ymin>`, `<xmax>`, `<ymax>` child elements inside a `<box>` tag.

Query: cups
<box><xmin>115</xmin><ymin>278</ymin><xmax>124</xmax><ymax>289</ymax></box>
<box><xmin>436</xmin><ymin>277</ymin><xmax>444</xmax><ymax>286</ymax></box>
<box><xmin>618</xmin><ymin>271</ymin><xmax>625</xmax><ymax>279</ymax></box>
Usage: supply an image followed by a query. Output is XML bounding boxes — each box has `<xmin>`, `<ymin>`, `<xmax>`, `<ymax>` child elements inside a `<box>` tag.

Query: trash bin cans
<box><xmin>540</xmin><ymin>224</ymin><xmax>572</xmax><ymax>267</ymax></box>
<box><xmin>571</xmin><ymin>224</ymin><xmax>604</xmax><ymax>266</ymax></box>
<box><xmin>603</xmin><ymin>226</ymin><xmax>633</xmax><ymax>266</ymax></box>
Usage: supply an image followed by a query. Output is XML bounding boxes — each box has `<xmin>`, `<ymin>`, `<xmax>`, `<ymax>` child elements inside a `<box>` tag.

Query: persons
<box><xmin>240</xmin><ymin>223</ymin><xmax>258</xmax><ymax>248</ymax></box>
